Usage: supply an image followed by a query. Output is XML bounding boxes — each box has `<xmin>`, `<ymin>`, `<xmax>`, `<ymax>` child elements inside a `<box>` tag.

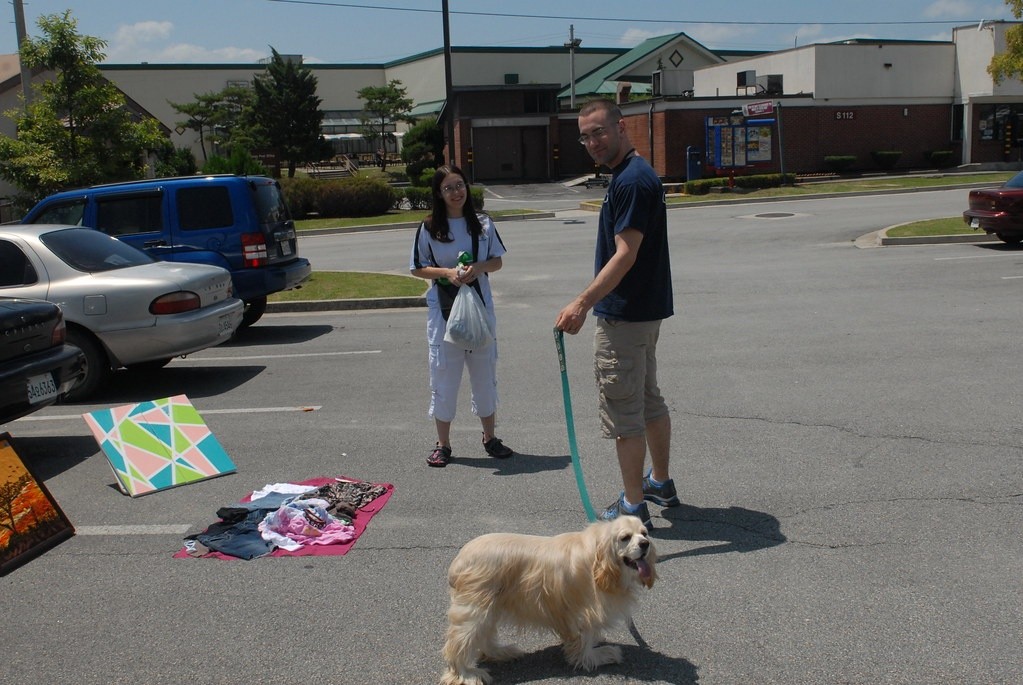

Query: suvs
<box><xmin>3</xmin><ymin>174</ymin><xmax>314</xmax><ymax>343</ymax></box>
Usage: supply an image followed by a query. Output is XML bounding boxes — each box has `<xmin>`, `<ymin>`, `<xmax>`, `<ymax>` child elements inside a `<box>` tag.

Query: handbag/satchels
<box><xmin>443</xmin><ymin>262</ymin><xmax>494</xmax><ymax>350</ymax></box>
<box><xmin>434</xmin><ymin>278</ymin><xmax>486</xmax><ymax>322</ymax></box>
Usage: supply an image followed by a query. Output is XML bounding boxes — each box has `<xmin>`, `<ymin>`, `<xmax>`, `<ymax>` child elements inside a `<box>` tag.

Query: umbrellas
<box><xmin>321</xmin><ymin>132</ymin><xmax>406</xmax><ymax>151</ymax></box>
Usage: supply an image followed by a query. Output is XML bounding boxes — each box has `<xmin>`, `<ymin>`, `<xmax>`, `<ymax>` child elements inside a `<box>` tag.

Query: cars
<box><xmin>0</xmin><ymin>224</ymin><xmax>245</xmax><ymax>397</ymax></box>
<box><xmin>0</xmin><ymin>299</ymin><xmax>88</xmax><ymax>425</ymax></box>
<box><xmin>964</xmin><ymin>173</ymin><xmax>1023</xmax><ymax>245</ymax></box>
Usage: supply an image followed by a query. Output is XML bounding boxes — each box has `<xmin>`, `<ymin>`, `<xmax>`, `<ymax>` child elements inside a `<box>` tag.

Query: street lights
<box><xmin>564</xmin><ymin>37</ymin><xmax>582</xmax><ymax>108</ymax></box>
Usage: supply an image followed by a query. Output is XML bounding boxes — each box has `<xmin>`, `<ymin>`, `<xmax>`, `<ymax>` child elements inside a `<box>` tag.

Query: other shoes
<box><xmin>481</xmin><ymin>431</ymin><xmax>514</xmax><ymax>458</ymax></box>
<box><xmin>426</xmin><ymin>442</ymin><xmax>452</xmax><ymax>466</ymax></box>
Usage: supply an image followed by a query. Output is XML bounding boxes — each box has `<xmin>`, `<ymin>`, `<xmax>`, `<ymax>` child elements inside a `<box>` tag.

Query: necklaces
<box><xmin>623</xmin><ymin>147</ymin><xmax>635</xmax><ymax>161</ymax></box>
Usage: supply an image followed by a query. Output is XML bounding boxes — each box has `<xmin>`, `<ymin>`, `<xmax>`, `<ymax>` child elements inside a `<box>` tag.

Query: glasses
<box><xmin>575</xmin><ymin>121</ymin><xmax>619</xmax><ymax>145</ymax></box>
<box><xmin>440</xmin><ymin>183</ymin><xmax>466</xmax><ymax>193</ymax></box>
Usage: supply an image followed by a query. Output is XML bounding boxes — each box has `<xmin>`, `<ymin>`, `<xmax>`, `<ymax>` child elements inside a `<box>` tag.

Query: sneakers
<box><xmin>642</xmin><ymin>467</ymin><xmax>680</xmax><ymax>507</ymax></box>
<box><xmin>597</xmin><ymin>489</ymin><xmax>654</xmax><ymax>531</ymax></box>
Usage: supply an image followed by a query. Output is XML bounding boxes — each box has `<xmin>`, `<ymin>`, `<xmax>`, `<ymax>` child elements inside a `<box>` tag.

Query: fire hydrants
<box><xmin>728</xmin><ymin>169</ymin><xmax>734</xmax><ymax>190</ymax></box>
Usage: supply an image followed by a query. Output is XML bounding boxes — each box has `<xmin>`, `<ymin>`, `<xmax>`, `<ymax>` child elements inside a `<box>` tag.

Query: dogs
<box><xmin>435</xmin><ymin>513</ymin><xmax>658</xmax><ymax>684</ymax></box>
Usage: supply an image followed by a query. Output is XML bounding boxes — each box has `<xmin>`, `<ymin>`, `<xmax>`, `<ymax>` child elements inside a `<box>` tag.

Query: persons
<box><xmin>376</xmin><ymin>147</ymin><xmax>385</xmax><ymax>167</ymax></box>
<box><xmin>409</xmin><ymin>165</ymin><xmax>514</xmax><ymax>465</ymax></box>
<box><xmin>554</xmin><ymin>101</ymin><xmax>680</xmax><ymax>535</ymax></box>
